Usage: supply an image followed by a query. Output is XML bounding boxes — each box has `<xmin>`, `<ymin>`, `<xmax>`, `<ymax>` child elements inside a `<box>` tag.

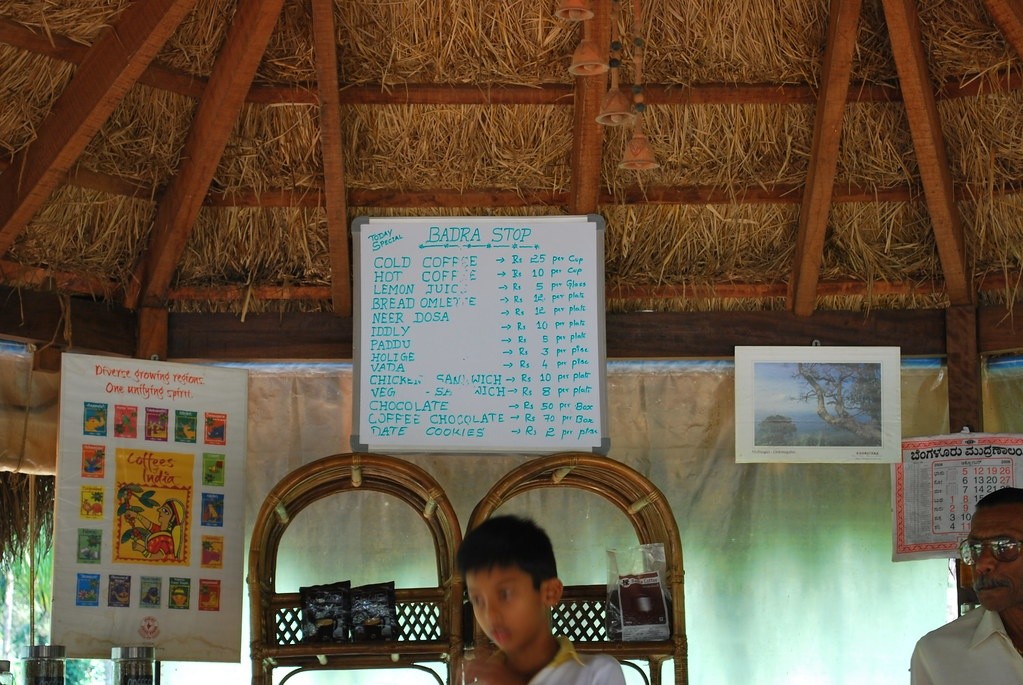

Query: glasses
<box><xmin>960</xmin><ymin>537</ymin><xmax>1023</xmax><ymax>566</ymax></box>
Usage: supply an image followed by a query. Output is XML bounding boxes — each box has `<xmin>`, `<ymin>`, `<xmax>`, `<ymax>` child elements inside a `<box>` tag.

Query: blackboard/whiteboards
<box><xmin>349</xmin><ymin>214</ymin><xmax>612</xmax><ymax>458</ymax></box>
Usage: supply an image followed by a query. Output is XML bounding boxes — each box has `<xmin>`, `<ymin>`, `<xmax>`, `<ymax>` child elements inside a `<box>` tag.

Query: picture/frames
<box><xmin>734</xmin><ymin>345</ymin><xmax>903</xmax><ymax>464</ymax></box>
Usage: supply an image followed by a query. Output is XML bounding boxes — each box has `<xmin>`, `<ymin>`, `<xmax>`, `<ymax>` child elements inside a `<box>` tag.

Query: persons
<box><xmin>457</xmin><ymin>515</ymin><xmax>627</xmax><ymax>685</ymax></box>
<box><xmin>907</xmin><ymin>486</ymin><xmax>1023</xmax><ymax>685</ymax></box>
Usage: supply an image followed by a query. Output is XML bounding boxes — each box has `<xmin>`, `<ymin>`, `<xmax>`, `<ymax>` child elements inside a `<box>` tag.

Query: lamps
<box><xmin>554</xmin><ymin>0</ymin><xmax>660</xmax><ymax>171</ymax></box>
